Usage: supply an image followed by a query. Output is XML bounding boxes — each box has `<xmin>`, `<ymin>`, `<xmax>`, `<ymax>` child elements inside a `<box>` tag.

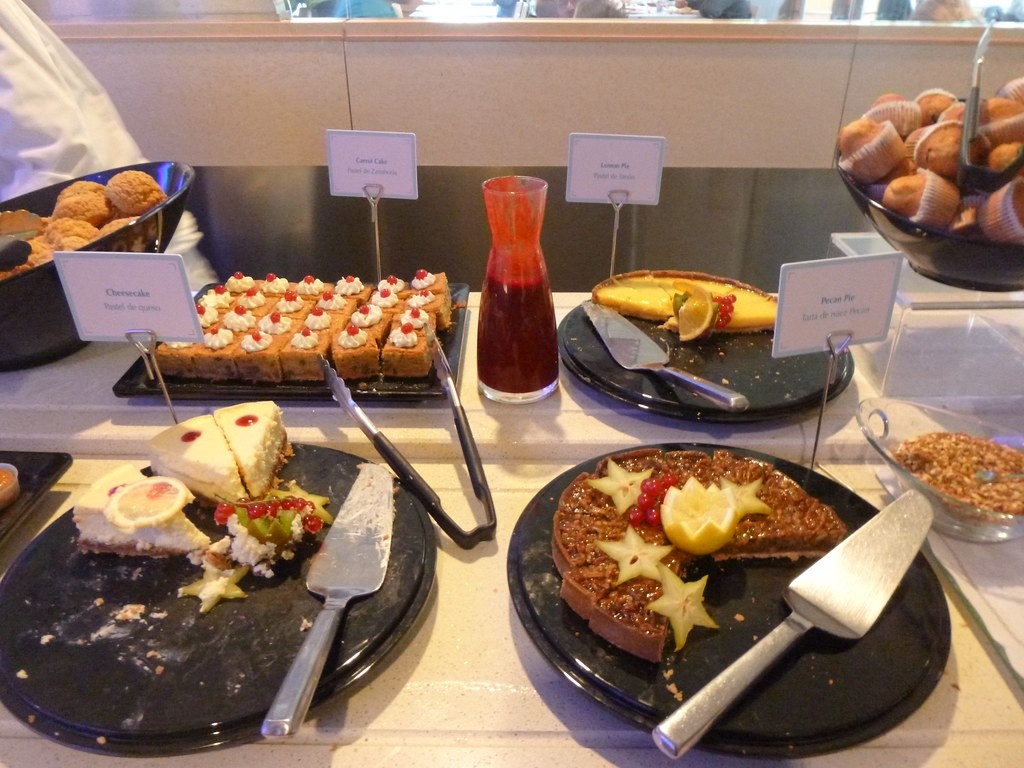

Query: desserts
<box><xmin>839</xmin><ymin>77</ymin><xmax>1024</xmax><ymax>244</ymax></box>
<box><xmin>153</xmin><ymin>269</ymin><xmax>451</xmax><ymax>381</ymax></box>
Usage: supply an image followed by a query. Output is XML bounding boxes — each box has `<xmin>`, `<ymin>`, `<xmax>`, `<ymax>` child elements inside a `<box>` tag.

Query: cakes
<box><xmin>72</xmin><ymin>400</ymin><xmax>334</xmax><ymax>613</ymax></box>
<box><xmin>552</xmin><ymin>449</ymin><xmax>847</xmax><ymax>660</ymax></box>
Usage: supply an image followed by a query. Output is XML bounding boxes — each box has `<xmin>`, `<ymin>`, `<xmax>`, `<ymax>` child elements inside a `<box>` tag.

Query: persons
<box><xmin>536</xmin><ymin>0</ymin><xmax>629</xmax><ymax>16</ymax></box>
<box><xmin>0</xmin><ymin>0</ymin><xmax>218</xmax><ymax>291</ymax></box>
<box><xmin>331</xmin><ymin>0</ymin><xmax>423</xmax><ymax>20</ymax></box>
<box><xmin>904</xmin><ymin>0</ymin><xmax>977</xmax><ymax>22</ymax></box>
<box><xmin>675</xmin><ymin>0</ymin><xmax>752</xmax><ymax>17</ymax></box>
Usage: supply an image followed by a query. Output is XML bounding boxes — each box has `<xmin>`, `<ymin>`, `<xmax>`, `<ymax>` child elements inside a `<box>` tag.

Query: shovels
<box><xmin>259</xmin><ymin>462</ymin><xmax>393</xmax><ymax>742</ymax></box>
<box><xmin>581</xmin><ymin>296</ymin><xmax>749</xmax><ymax>414</ymax></box>
<box><xmin>652</xmin><ymin>486</ymin><xmax>934</xmax><ymax>760</ymax></box>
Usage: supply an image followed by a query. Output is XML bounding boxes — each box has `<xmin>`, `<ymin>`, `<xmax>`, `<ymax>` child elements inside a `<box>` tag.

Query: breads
<box><xmin>0</xmin><ymin>170</ymin><xmax>167</xmax><ymax>279</ymax></box>
<box><xmin>0</xmin><ymin>468</ymin><xmax>19</xmax><ymax>508</ymax></box>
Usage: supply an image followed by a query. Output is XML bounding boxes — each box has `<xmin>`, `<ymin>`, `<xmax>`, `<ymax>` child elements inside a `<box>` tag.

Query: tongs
<box><xmin>959</xmin><ymin>22</ymin><xmax>1024</xmax><ymax>189</ymax></box>
<box><xmin>315</xmin><ymin>320</ymin><xmax>499</xmax><ymax>550</ymax></box>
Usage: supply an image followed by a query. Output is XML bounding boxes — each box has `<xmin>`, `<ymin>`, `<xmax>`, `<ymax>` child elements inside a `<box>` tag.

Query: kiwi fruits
<box><xmin>672</xmin><ymin>294</ymin><xmax>718</xmax><ymax>327</ymax></box>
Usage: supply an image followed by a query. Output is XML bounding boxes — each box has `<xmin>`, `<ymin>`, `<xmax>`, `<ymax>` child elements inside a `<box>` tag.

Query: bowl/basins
<box><xmin>854</xmin><ymin>394</ymin><xmax>1024</xmax><ymax>541</ymax></box>
<box><xmin>835</xmin><ymin>137</ymin><xmax>1024</xmax><ymax>293</ymax></box>
<box><xmin>1</xmin><ymin>162</ymin><xmax>196</xmax><ymax>372</ymax></box>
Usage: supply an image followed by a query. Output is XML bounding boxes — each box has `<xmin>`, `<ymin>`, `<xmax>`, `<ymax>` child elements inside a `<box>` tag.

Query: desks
<box><xmin>0</xmin><ymin>293</ymin><xmax>1024</xmax><ymax>768</ymax></box>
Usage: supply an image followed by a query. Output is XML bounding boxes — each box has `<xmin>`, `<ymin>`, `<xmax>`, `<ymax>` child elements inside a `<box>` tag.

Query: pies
<box><xmin>592</xmin><ymin>269</ymin><xmax>778</xmax><ymax>333</ymax></box>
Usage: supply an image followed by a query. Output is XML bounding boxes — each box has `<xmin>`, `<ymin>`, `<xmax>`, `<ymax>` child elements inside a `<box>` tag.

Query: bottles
<box><xmin>478</xmin><ymin>177</ymin><xmax>562</xmax><ymax>404</ymax></box>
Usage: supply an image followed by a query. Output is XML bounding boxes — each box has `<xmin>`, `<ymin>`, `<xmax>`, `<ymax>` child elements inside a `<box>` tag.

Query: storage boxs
<box><xmin>825</xmin><ymin>234</ymin><xmax>1024</xmax><ymax>400</ymax></box>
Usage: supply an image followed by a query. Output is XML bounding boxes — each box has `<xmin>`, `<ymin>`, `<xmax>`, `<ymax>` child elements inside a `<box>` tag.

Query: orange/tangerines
<box><xmin>674</xmin><ymin>281</ymin><xmax>712</xmax><ymax>342</ymax></box>
